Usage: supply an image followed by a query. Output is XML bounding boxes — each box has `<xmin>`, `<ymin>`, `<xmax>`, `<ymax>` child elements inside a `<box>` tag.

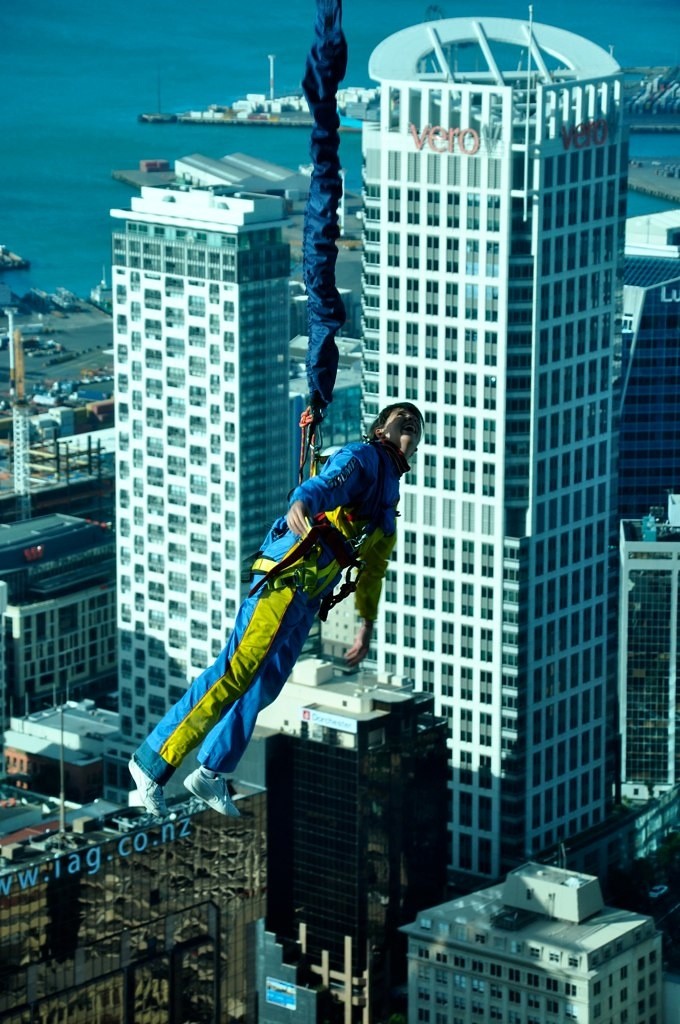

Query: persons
<box><xmin>127</xmin><ymin>402</ymin><xmax>426</xmax><ymax>818</ymax></box>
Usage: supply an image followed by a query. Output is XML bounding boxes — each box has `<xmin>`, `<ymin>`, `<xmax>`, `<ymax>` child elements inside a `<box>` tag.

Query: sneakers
<box><xmin>128</xmin><ymin>758</ymin><xmax>171</xmax><ymax>818</ymax></box>
<box><xmin>181</xmin><ymin>767</ymin><xmax>243</xmax><ymax>817</ymax></box>
